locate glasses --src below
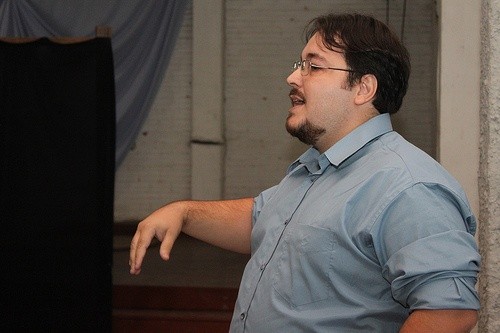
[293,60,365,76]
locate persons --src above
[128,13,482,333]
